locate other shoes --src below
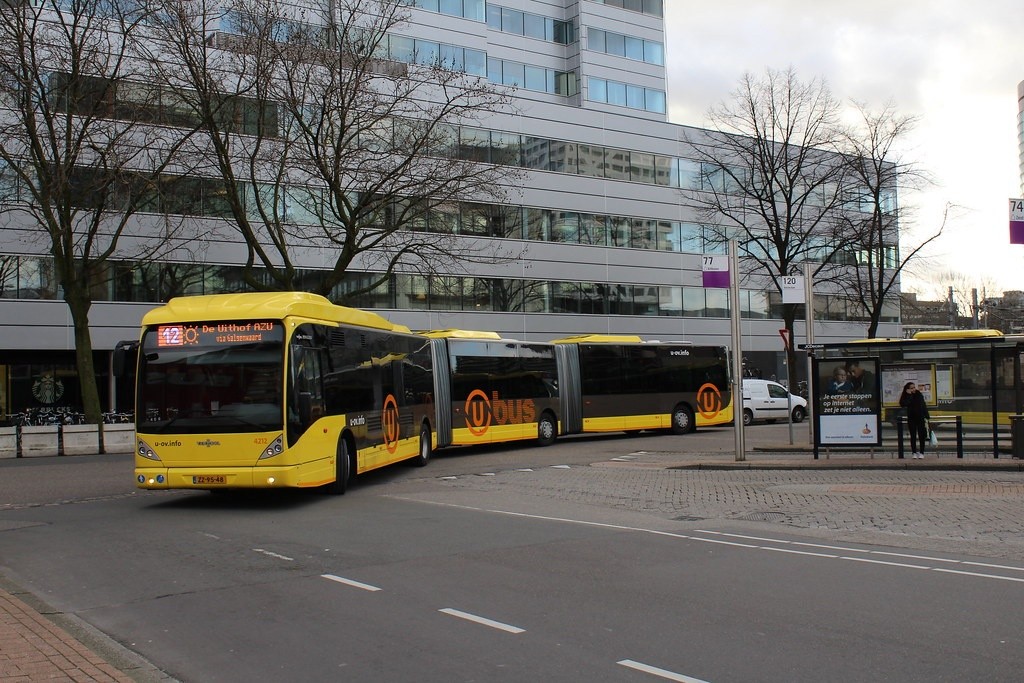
[918,453,924,458]
[912,453,917,458]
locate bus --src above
[848,329,1024,428]
[113,292,736,495]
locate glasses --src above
[838,374,846,376]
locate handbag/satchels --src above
[928,421,938,448]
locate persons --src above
[845,361,876,414]
[899,382,930,459]
[828,365,852,395]
[771,374,776,382]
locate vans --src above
[742,379,808,425]
